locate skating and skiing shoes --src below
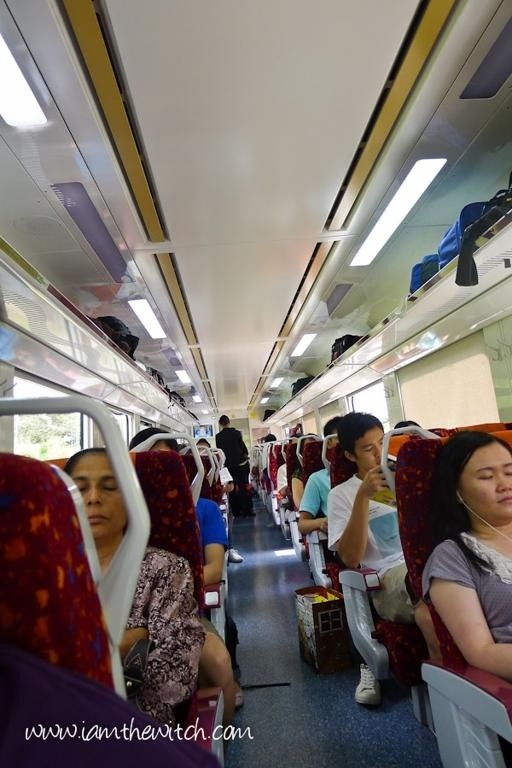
[228,548,244,563]
[353,660,386,707]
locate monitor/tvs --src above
[193,424,214,439]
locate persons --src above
[51,408,512,744]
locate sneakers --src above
[233,511,257,517]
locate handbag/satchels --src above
[293,584,347,675]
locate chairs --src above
[0,395,512,768]
